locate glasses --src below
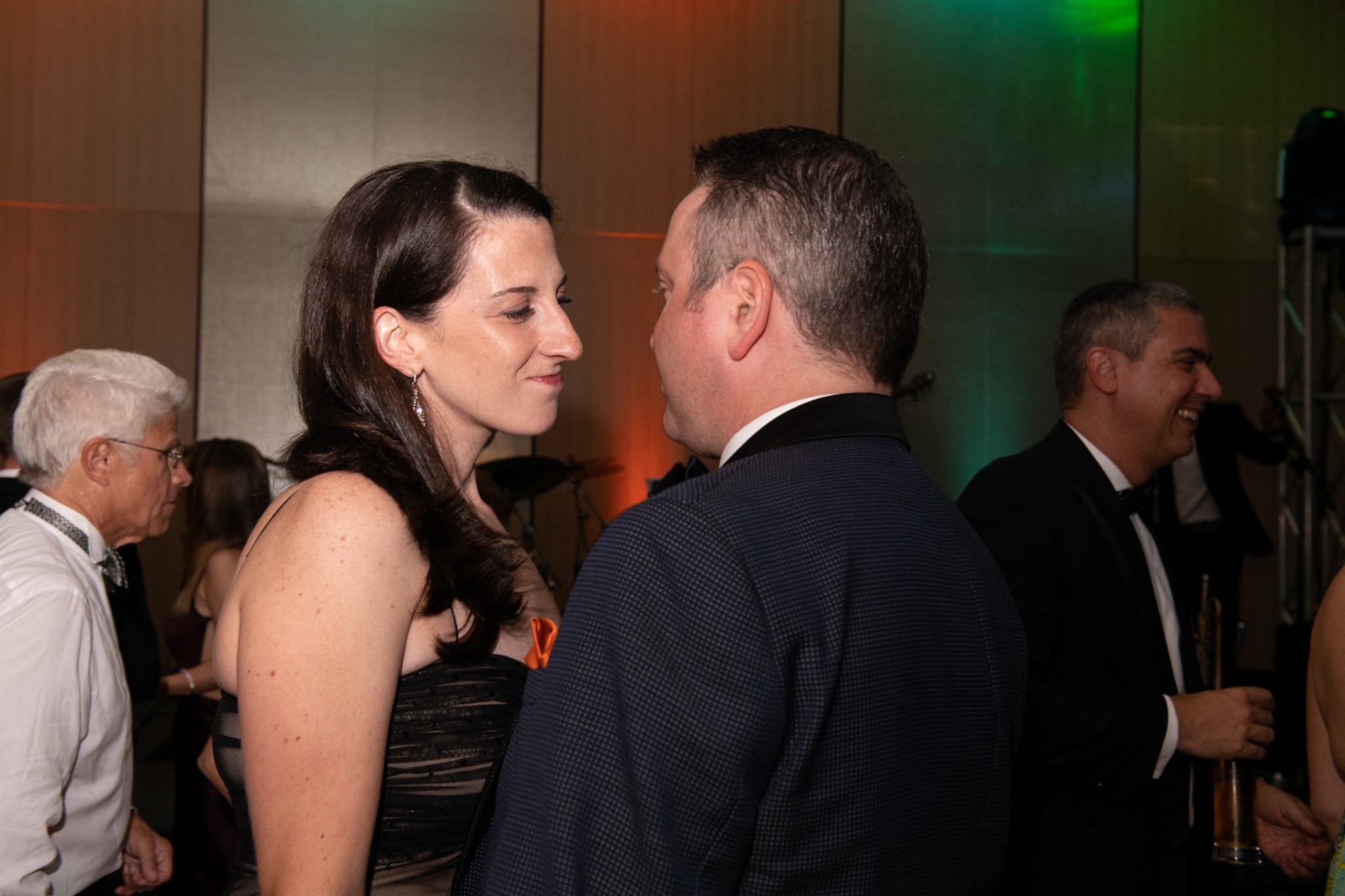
[107,436,184,469]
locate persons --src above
[466,122,1002,896]
[177,158,588,896]
[958,278,1345,896]
[0,350,190,895]
[157,437,274,895]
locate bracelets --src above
[182,666,196,692]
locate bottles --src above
[1211,756,1264,866]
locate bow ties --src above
[647,456,710,499]
[16,497,128,588]
[1116,479,1156,515]
